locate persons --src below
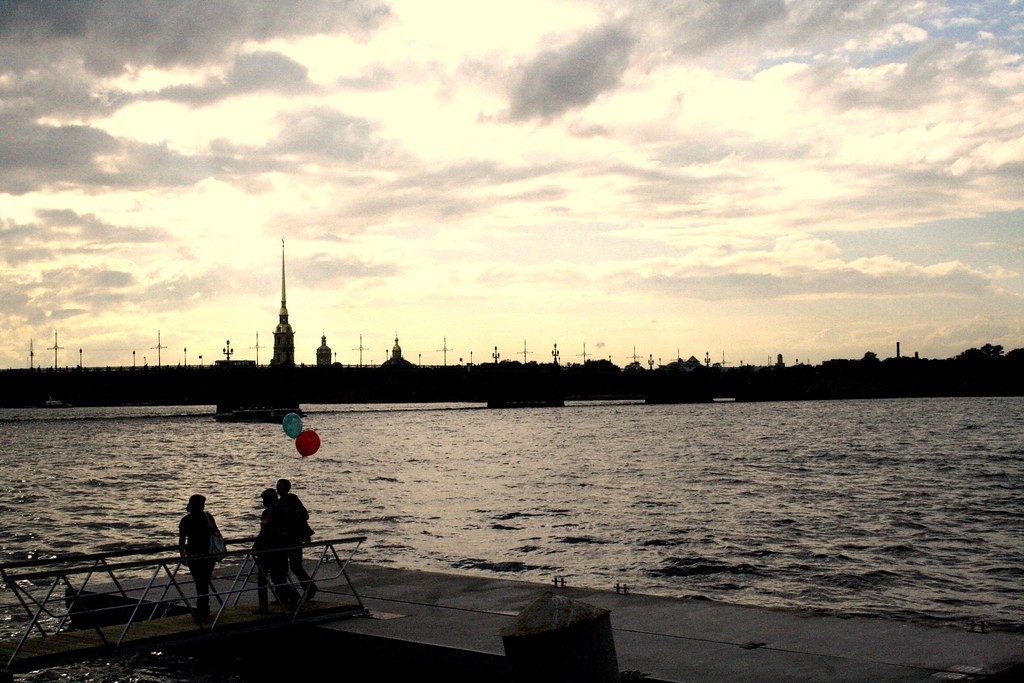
[179,494,220,616]
[251,479,318,615]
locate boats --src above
[213,338,306,422]
[45,397,72,408]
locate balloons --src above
[283,413,303,439]
[295,430,321,457]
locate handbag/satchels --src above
[209,531,226,561]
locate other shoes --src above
[302,589,319,600]
[271,599,289,605]
[254,609,269,615]
